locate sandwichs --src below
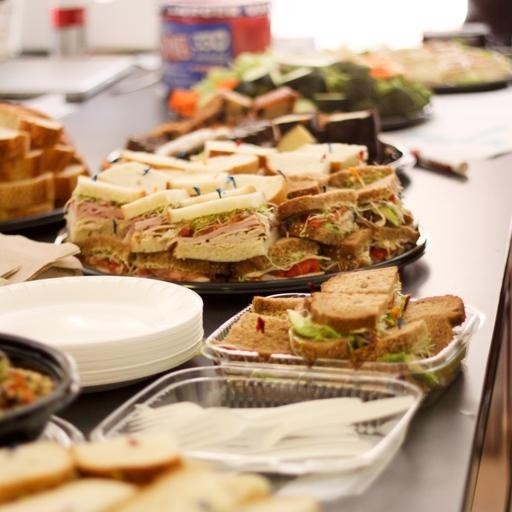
[0,98,465,377]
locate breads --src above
[0,430,323,511]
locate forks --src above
[125,394,415,460]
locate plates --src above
[171,104,428,130]
[121,137,415,177]
[0,275,204,386]
[353,44,511,94]
[51,229,428,290]
[0,200,66,236]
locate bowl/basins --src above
[0,333,81,449]
